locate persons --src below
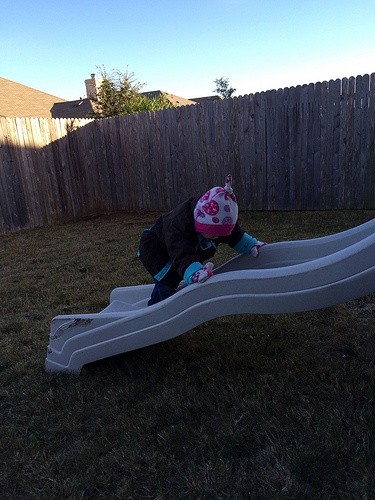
[138,173,268,307]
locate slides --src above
[44,215,375,378]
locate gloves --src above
[191,261,215,283]
[250,240,268,257]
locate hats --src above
[193,174,239,236]
